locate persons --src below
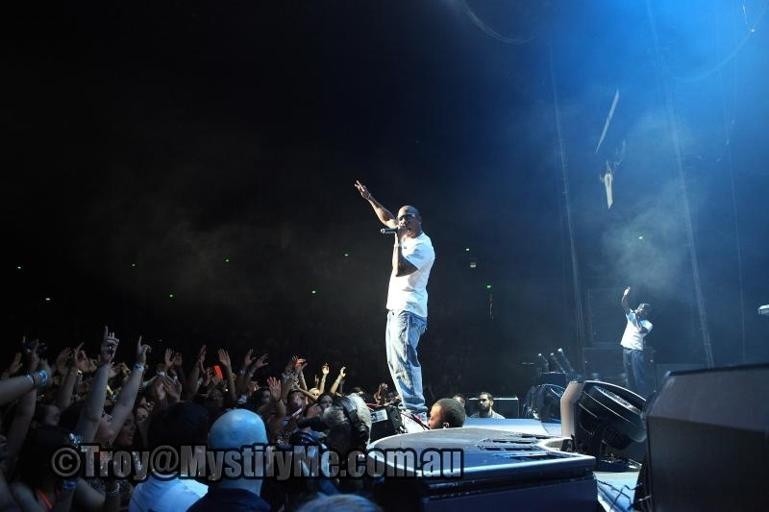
[354,179,436,423]
[1,320,507,510]
[620,286,654,394]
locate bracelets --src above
[396,243,404,248]
[365,192,372,200]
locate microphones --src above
[380,227,398,234]
[631,311,635,314]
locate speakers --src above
[645,361,769,512]
